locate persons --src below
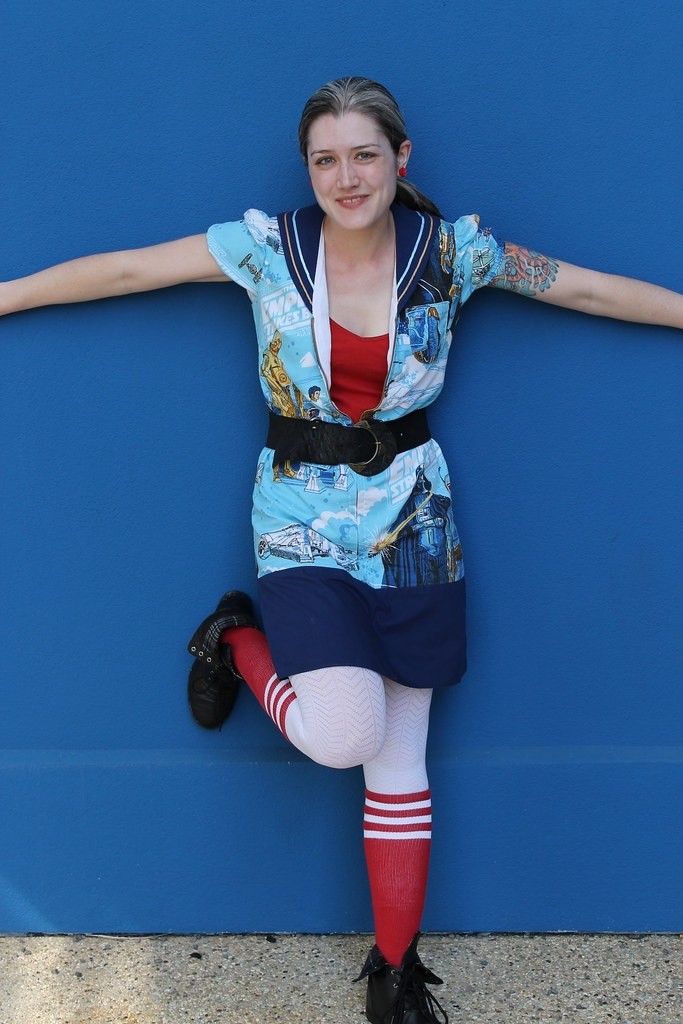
[0,76,682,1024]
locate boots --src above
[350,930,450,1024]
[188,589,264,732]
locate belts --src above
[264,408,431,476]
